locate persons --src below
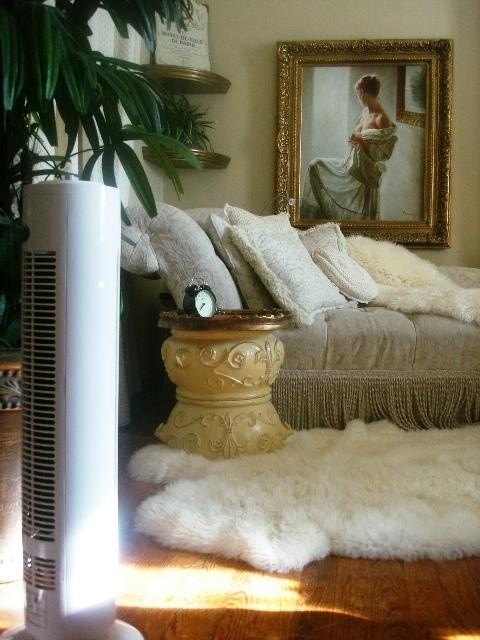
[300,75,398,221]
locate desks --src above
[149,305,300,458]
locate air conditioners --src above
[1,180,154,640]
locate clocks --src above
[181,279,219,318]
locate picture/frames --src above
[271,34,455,254]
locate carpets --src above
[123,419,478,574]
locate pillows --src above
[206,204,376,325]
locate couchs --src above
[118,205,478,431]
